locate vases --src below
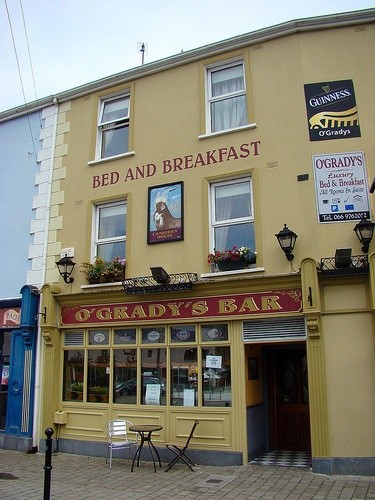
[88,272,124,285]
[217,261,247,270]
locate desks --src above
[129,425,163,473]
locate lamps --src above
[353,214,375,253]
[34,306,47,323]
[335,249,352,268]
[55,253,76,283]
[275,224,298,261]
[150,267,170,284]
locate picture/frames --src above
[147,181,184,245]
[248,357,259,380]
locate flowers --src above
[79,256,126,282]
[208,245,257,264]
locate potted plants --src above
[68,382,109,404]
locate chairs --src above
[105,419,140,470]
[165,420,199,473]
